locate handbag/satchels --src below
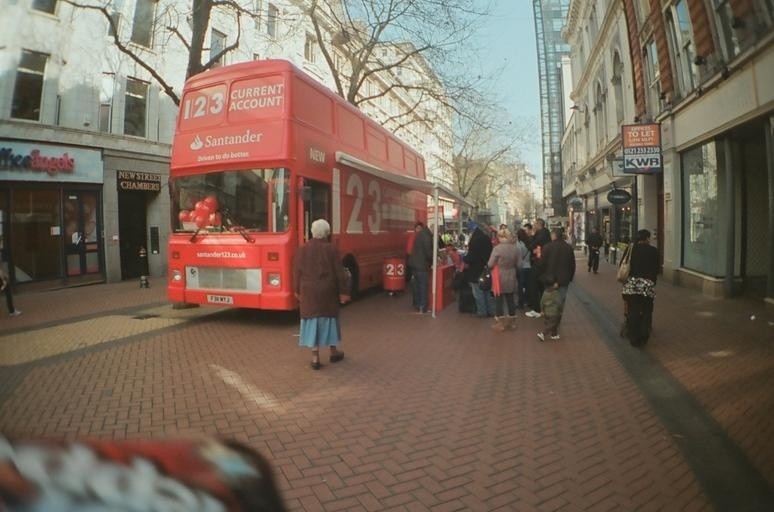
[616,263,631,282]
[478,267,491,290]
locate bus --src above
[165,58,427,315]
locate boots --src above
[504,314,517,330]
[490,315,505,331]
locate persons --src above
[408,217,576,341]
[619,230,659,345]
[585,226,602,272]
[294,218,349,368]
[1,238,21,318]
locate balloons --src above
[176,196,224,229]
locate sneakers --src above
[330,352,343,362]
[524,310,542,319]
[8,309,22,318]
[311,360,320,370]
